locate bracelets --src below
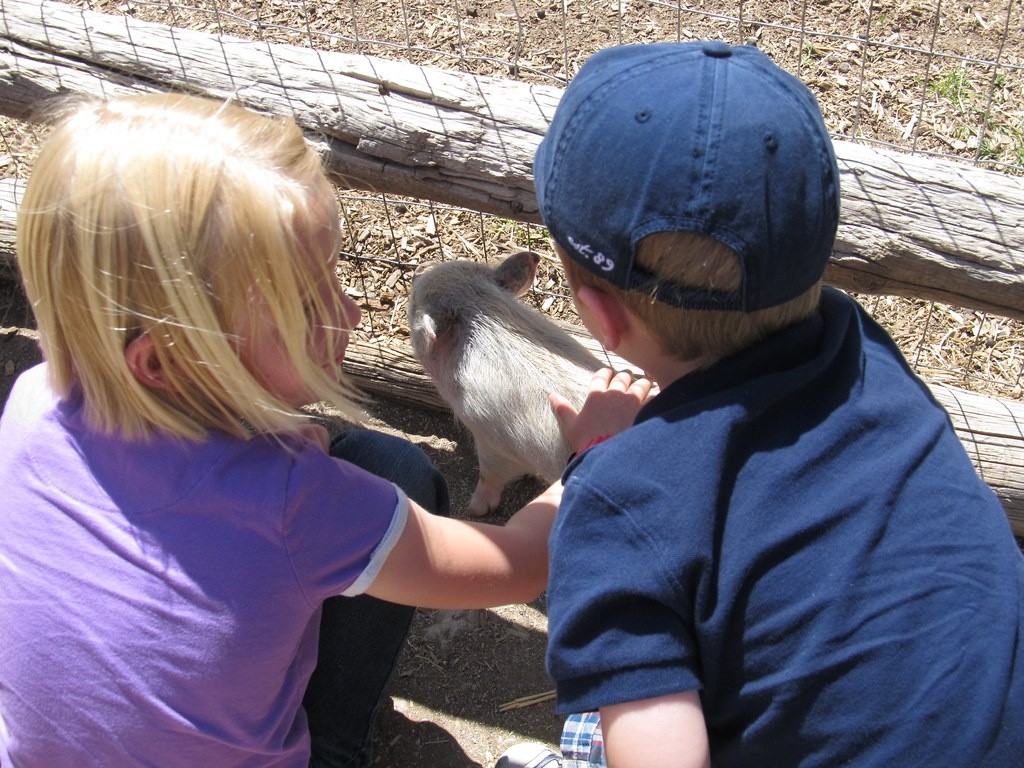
[567,433,614,459]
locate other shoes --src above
[495,742,562,768]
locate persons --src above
[496,40,1024,768]
[0,90,659,768]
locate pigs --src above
[406,252,664,517]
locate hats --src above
[532,39,839,311]
